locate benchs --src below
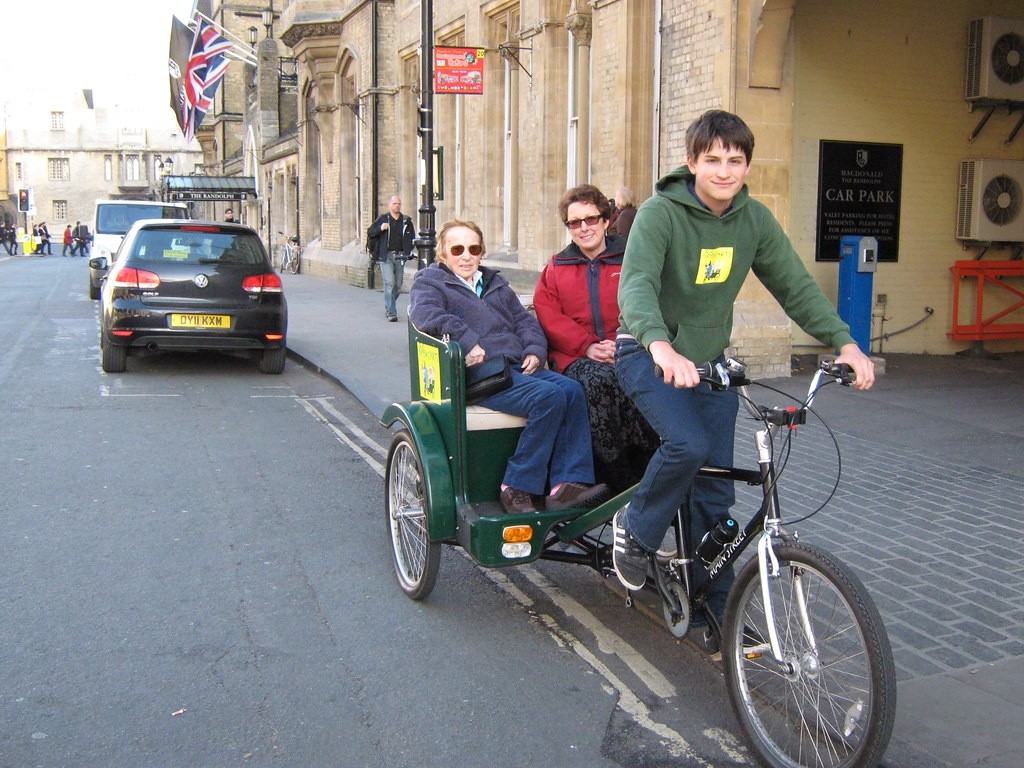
[464,404,531,432]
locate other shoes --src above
[71,254,77,257]
[62,255,67,257]
[34,252,46,254]
[81,255,86,257]
[48,253,53,255]
[10,253,18,256]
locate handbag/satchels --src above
[34,233,43,244]
[465,353,513,399]
[366,213,390,259]
[38,227,47,240]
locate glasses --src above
[451,245,483,256]
[566,214,603,229]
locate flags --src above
[169,13,233,144]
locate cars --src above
[88,218,288,374]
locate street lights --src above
[164,157,173,203]
[158,162,165,203]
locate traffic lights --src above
[20,188,29,212]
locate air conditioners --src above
[966,13,1024,103]
[957,158,1024,241]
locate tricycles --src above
[379,308,899,768]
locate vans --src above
[88,198,191,301]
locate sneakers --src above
[703,624,772,662]
[389,316,397,322]
[500,486,536,514]
[613,502,648,591]
[545,482,608,510]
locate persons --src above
[224,208,240,223]
[0,221,89,257]
[368,196,416,323]
[611,110,875,663]
[407,216,597,514]
[531,185,662,498]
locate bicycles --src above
[278,231,301,275]
